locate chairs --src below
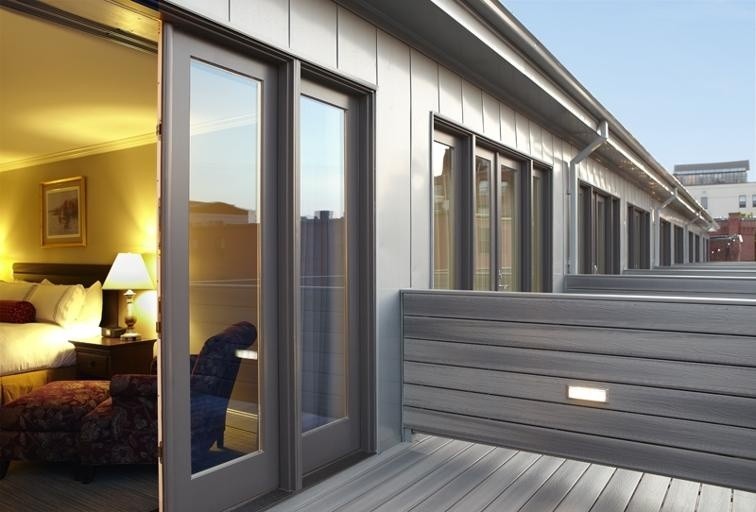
[78,322,254,485]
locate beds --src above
[0,263,121,413]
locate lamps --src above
[102,252,154,341]
[566,385,609,404]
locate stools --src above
[0,380,112,480]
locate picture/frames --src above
[38,175,87,249]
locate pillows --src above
[0,279,105,327]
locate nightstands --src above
[68,337,156,381]
